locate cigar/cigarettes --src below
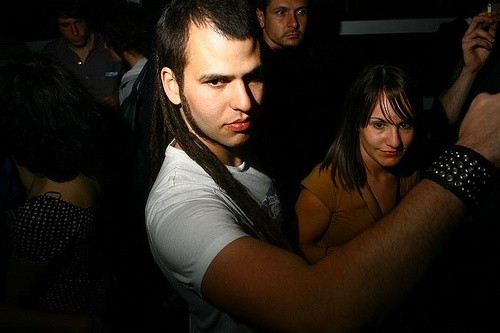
[487,3,492,18]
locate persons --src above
[0,0,500,333]
